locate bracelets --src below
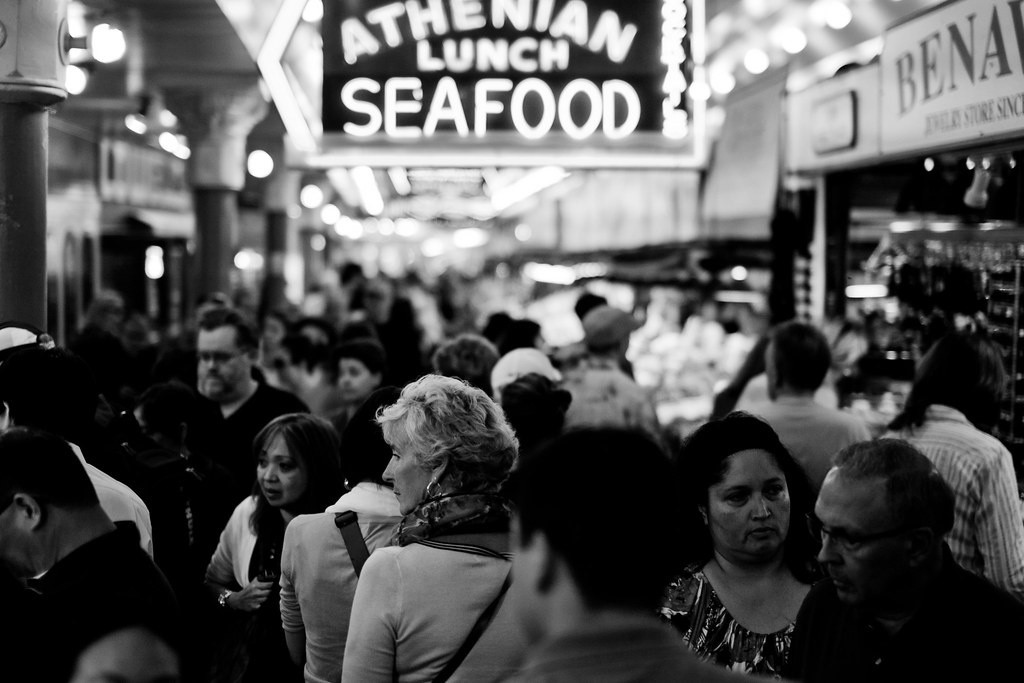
[218,590,233,607]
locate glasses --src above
[804,510,902,552]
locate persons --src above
[0,263,1024,683]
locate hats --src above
[583,304,642,348]
[489,345,565,402]
